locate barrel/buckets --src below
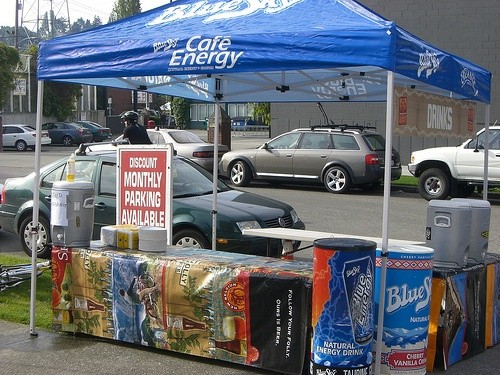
[50,180,95,247]
[426,199,472,269]
[451,196,492,265]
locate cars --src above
[2,124,52,151]
[75,121,112,142]
[0,151,305,259]
[113,127,228,173]
[41,122,93,146]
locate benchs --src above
[337,142,356,148]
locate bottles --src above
[209,339,247,357]
[75,296,104,311]
[166,315,206,332]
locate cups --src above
[223,316,246,340]
[66,159,75,182]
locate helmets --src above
[120,111,139,119]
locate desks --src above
[426,253,500,372]
[242,226,427,258]
[50,238,314,375]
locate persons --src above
[120,110,154,145]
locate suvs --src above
[217,124,402,193]
[407,124,500,201]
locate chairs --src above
[319,141,328,148]
[303,138,312,148]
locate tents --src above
[29,0,492,375]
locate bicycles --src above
[133,274,159,320]
